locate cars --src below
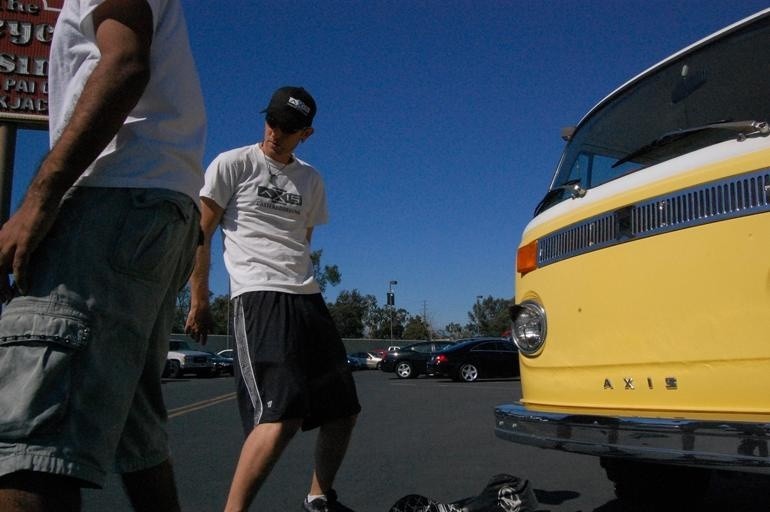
[346,337,520,383]
[164,337,234,378]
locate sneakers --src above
[302,490,357,512]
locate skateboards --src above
[389,472,539,512]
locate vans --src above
[493,6,770,502]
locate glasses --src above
[264,114,306,134]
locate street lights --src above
[386,278,398,346]
[476,293,483,337]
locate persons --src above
[0,0,209,512]
[186,87,363,511]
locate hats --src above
[260,86,317,125]
